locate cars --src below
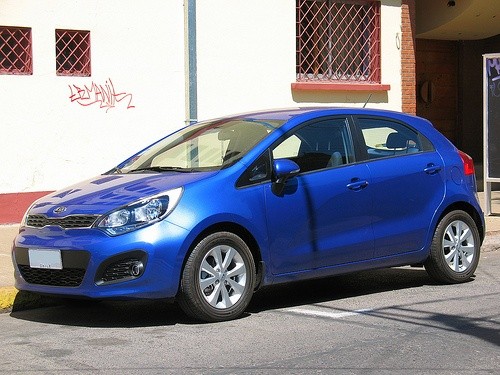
[12,107,487,323]
